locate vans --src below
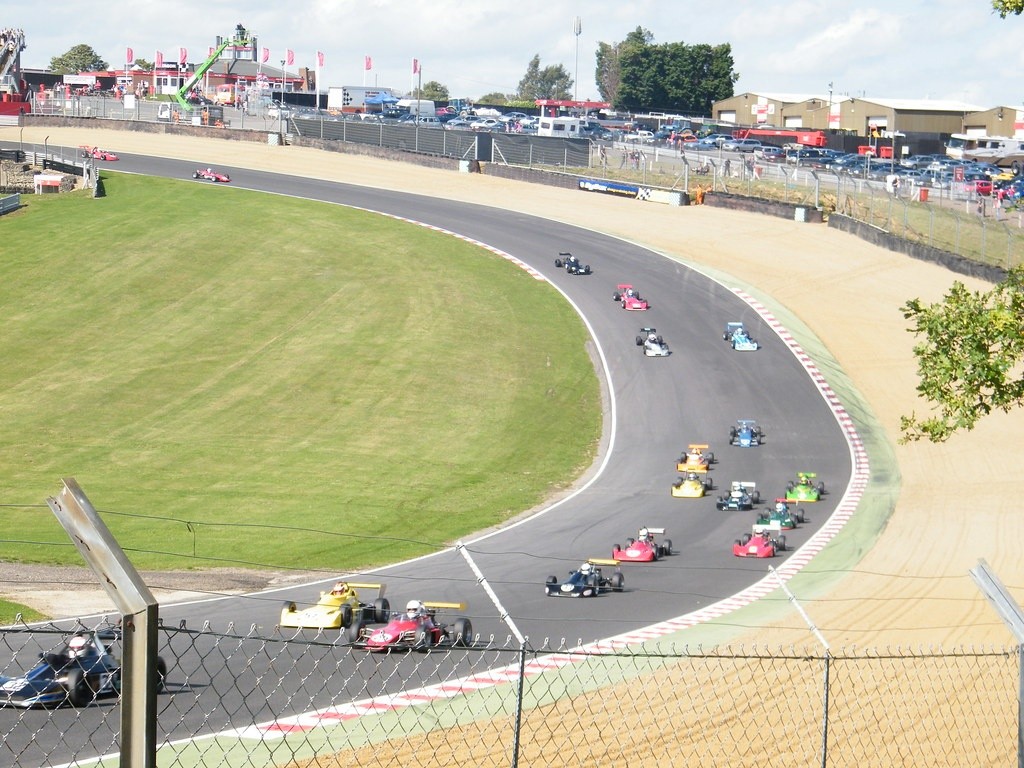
[395,99,435,118]
[723,139,761,152]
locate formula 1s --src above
[281,583,392,631]
[636,327,671,356]
[670,466,713,498]
[192,169,231,182]
[545,557,626,597]
[756,497,807,529]
[716,481,760,510]
[79,145,119,161]
[1,626,166,707]
[611,526,673,562]
[677,443,714,472]
[556,251,591,276]
[612,283,647,310]
[346,600,474,653]
[727,419,762,447]
[785,472,826,501]
[731,523,787,559]
[722,322,758,351]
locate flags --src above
[318,51,324,68]
[157,52,163,67]
[413,58,418,73]
[365,56,371,69]
[262,48,269,62]
[287,50,294,65]
[210,48,214,57]
[180,48,187,64]
[127,48,133,63]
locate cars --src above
[436,108,540,134]
[268,99,291,111]
[185,95,214,106]
[378,108,404,120]
[394,114,422,124]
[212,96,234,107]
[579,120,1024,201]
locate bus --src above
[218,84,242,108]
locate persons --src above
[332,582,352,597]
[580,563,594,576]
[628,288,634,295]
[670,418,812,537]
[638,528,649,543]
[570,256,575,262]
[207,168,212,175]
[203,107,208,125]
[648,332,657,341]
[723,158,731,176]
[736,328,745,334]
[67,637,90,658]
[893,177,899,198]
[172,109,179,125]
[405,600,423,621]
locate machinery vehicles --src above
[154,28,254,127]
[731,129,828,148]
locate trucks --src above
[942,134,1024,166]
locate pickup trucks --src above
[786,149,832,168]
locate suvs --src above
[753,146,783,158]
[403,115,441,129]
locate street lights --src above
[280,60,287,105]
[891,130,906,175]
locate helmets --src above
[692,449,697,454]
[742,425,748,432]
[800,476,808,484]
[68,636,89,657]
[755,528,763,537]
[207,168,212,173]
[776,503,783,512]
[649,334,656,341]
[639,528,648,541]
[732,481,741,491]
[333,581,349,596]
[737,328,743,334]
[406,600,421,618]
[688,472,697,480]
[570,256,576,262]
[581,563,591,575]
[628,290,634,296]
[97,148,102,153]
